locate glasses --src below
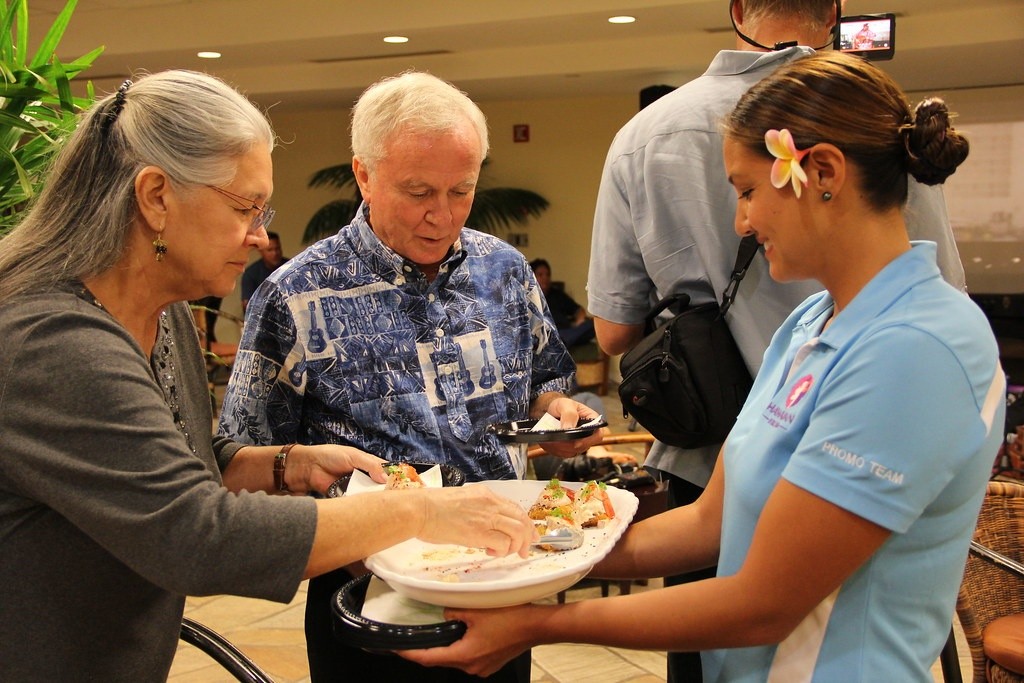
[205,183,275,233]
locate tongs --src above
[526,528,582,550]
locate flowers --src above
[764,128,810,199]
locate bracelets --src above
[273,444,295,493]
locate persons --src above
[241,232,290,313]
[0,70,543,683]
[531,258,594,350]
[586,0,967,683]
[393,50,1007,683]
[855,23,876,48]
[217,67,611,683]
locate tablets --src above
[833,13,896,61]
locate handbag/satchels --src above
[618,295,755,448]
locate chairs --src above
[189,305,244,419]
[551,281,609,397]
[953,481,1024,683]
[527,433,658,604]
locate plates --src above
[330,573,468,656]
[487,417,608,443]
[327,462,465,508]
[363,479,639,607]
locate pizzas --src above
[528,479,576,520]
[385,462,422,491]
[572,479,615,528]
